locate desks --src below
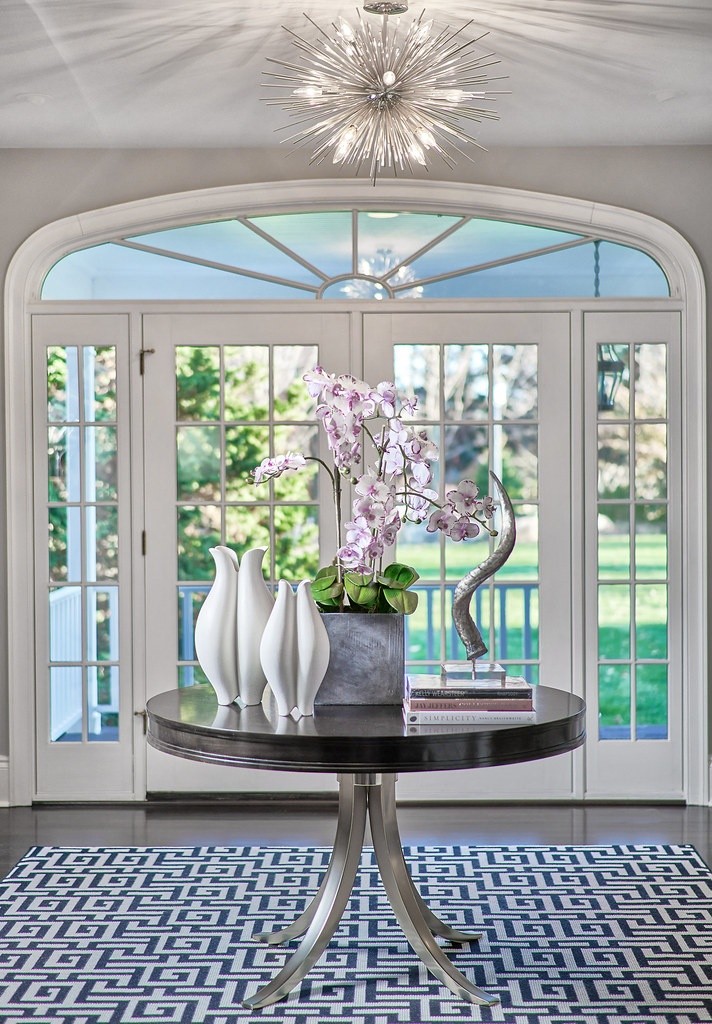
[145,683,586,1011]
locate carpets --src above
[0,843,712,1024]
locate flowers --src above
[250,364,499,615]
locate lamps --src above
[259,0,512,187]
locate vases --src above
[259,578,330,717]
[314,613,407,705]
[194,546,275,705]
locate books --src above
[401,674,536,726]
